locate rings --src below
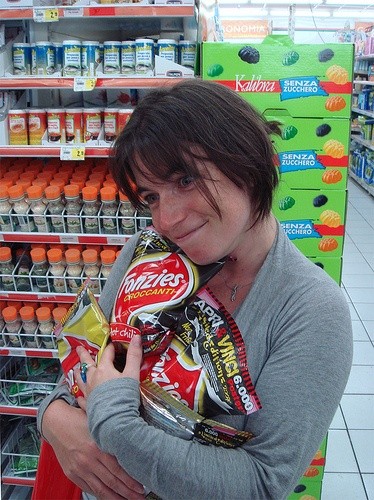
[78,362,94,384]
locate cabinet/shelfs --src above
[0,0,374,500]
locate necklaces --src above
[219,273,253,301]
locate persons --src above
[37,79,354,500]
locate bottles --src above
[0,156,152,235]
[0,240,124,295]
[0,300,74,349]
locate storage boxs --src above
[199,40,355,119]
[306,257,343,289]
[285,481,323,500]
[301,431,328,481]
[263,116,351,190]
[270,188,349,257]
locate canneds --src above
[12,38,198,76]
[7,107,135,144]
